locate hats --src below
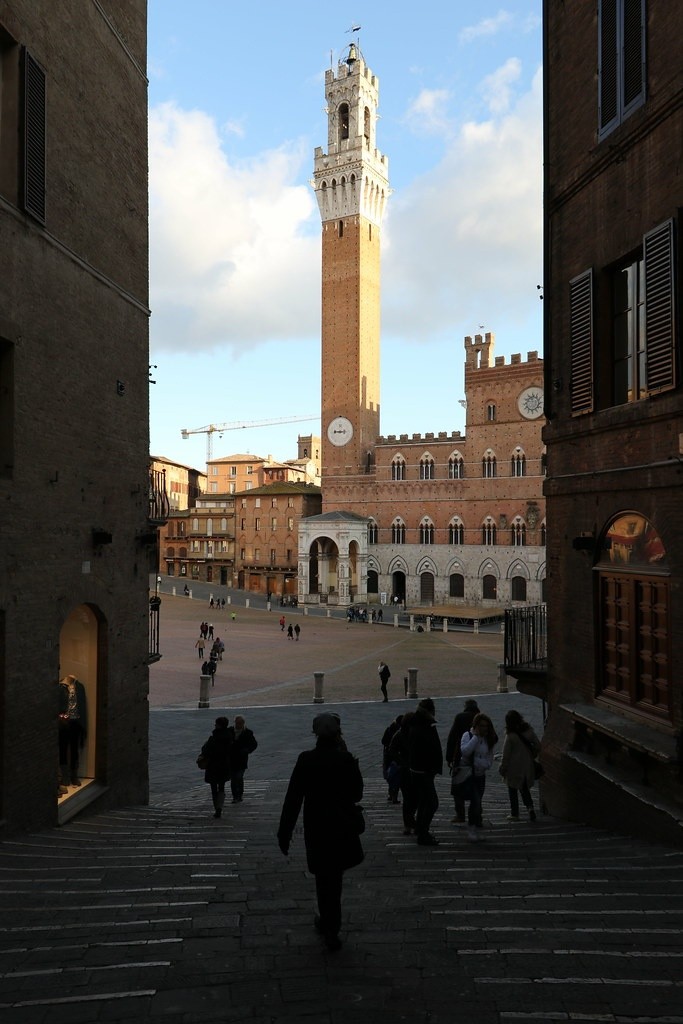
[313,710,341,736]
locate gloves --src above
[278,837,290,855]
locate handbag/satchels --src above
[355,803,366,834]
[534,758,546,780]
[456,773,480,801]
[196,754,205,768]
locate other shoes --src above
[313,917,342,954]
[417,836,439,845]
[451,816,466,822]
[71,778,81,786]
[61,777,70,786]
[387,797,401,804]
[231,797,243,803]
[507,814,518,821]
[213,807,223,818]
[528,809,536,823]
[58,789,68,798]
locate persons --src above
[276,711,364,939]
[266,591,300,642]
[430,612,436,627]
[377,662,389,702]
[184,584,237,687]
[380,698,540,845]
[345,593,404,622]
[203,716,232,819]
[59,675,88,796]
[226,715,258,804]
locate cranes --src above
[181,414,322,493]
